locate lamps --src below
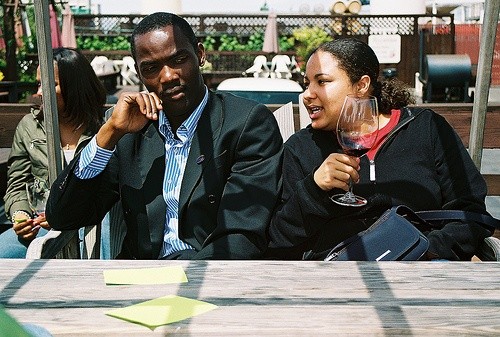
[418,28,474,103]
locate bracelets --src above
[12,211,31,225]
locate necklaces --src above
[67,122,84,150]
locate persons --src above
[264,36,495,261]
[0,47,107,259]
[44,12,285,260]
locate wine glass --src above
[331,94,379,206]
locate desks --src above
[0,257,500,337]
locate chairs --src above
[90,55,139,85]
[245,54,294,80]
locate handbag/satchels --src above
[324,210,430,261]
